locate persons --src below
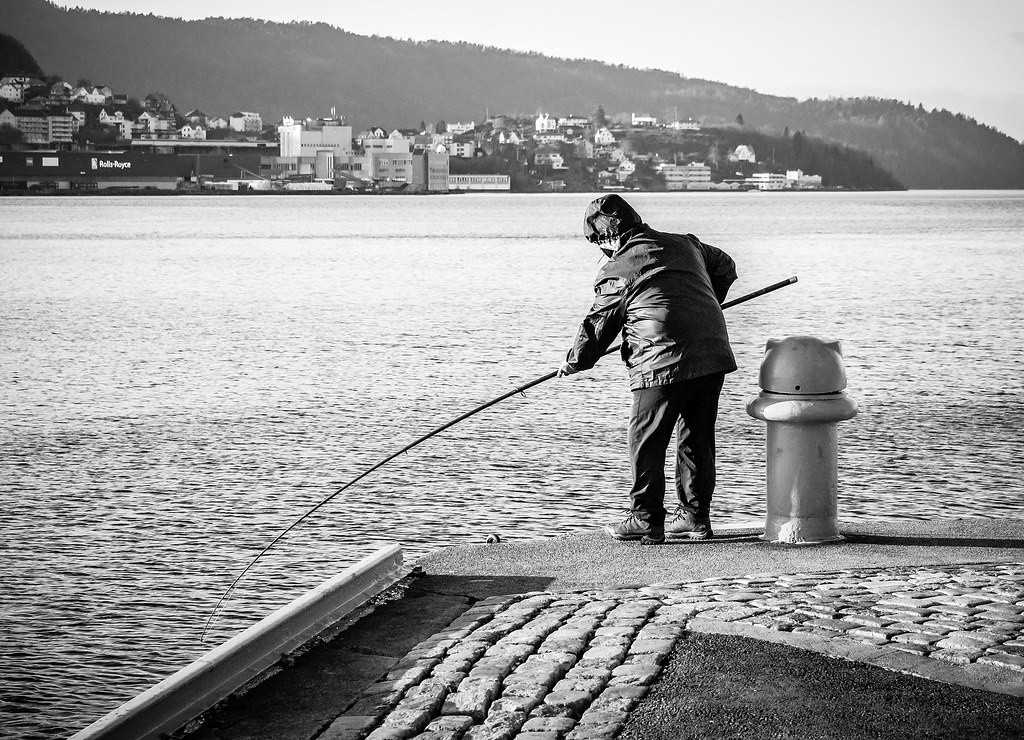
[557,193,737,545]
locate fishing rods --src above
[200,275,799,649]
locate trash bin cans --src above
[745,335,858,544]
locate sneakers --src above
[606,509,665,545]
[663,507,713,540]
